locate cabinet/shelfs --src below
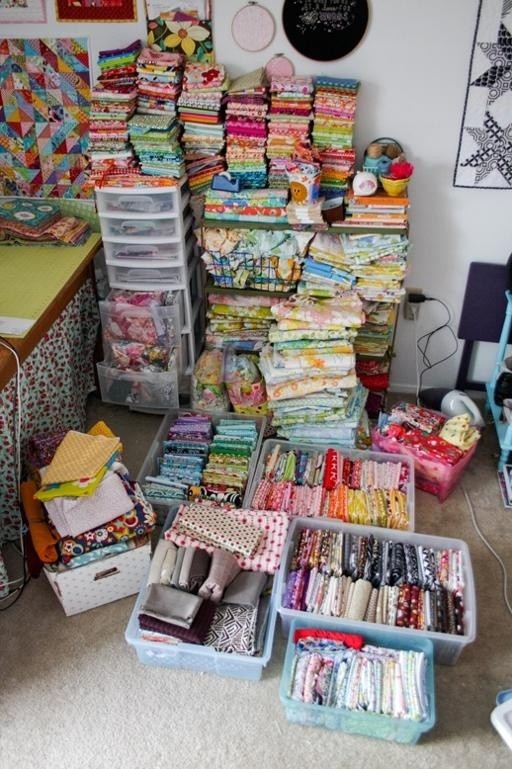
[481,288,512,474]
[96,173,412,419]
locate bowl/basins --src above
[378,174,410,197]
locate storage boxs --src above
[137,408,268,510]
[275,518,482,665]
[247,437,419,532]
[122,504,293,681]
[371,404,481,503]
[279,616,439,745]
[22,470,151,617]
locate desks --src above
[0,232,101,396]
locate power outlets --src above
[403,287,422,319]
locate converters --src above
[407,292,425,303]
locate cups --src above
[285,159,323,206]
[320,195,346,224]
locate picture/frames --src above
[1,0,212,24]
